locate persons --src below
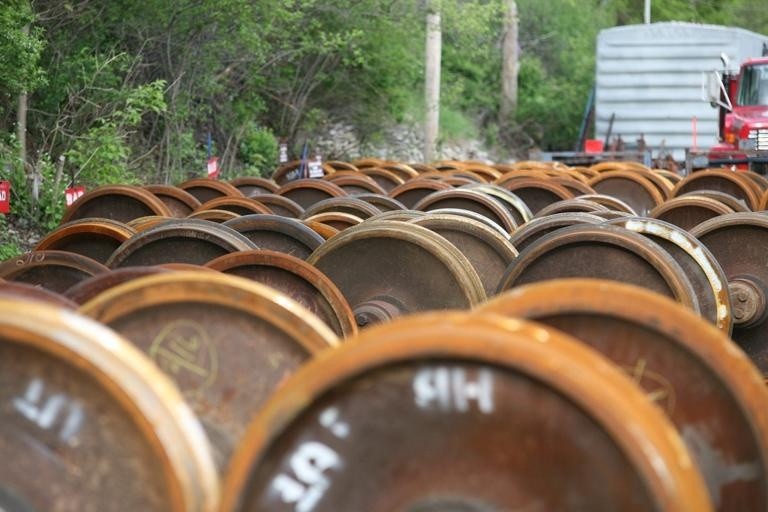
[651,155,686,179]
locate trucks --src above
[702,51,768,173]
